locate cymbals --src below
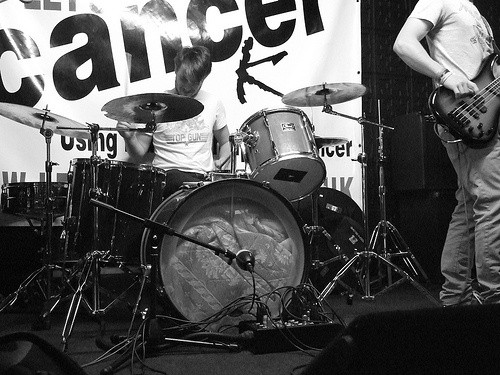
[314,137,348,147]
[282,83,366,107]
[101,92,204,123]
[0,102,92,139]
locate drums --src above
[62,158,166,268]
[141,177,310,333]
[238,106,326,201]
[1,182,71,215]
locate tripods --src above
[0,117,241,375]
[302,92,445,318]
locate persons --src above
[392,0,500,305]
[115,46,233,203]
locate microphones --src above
[236,249,255,272]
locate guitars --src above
[429,53,500,149]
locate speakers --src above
[301,302,500,375]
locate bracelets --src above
[435,68,452,85]
